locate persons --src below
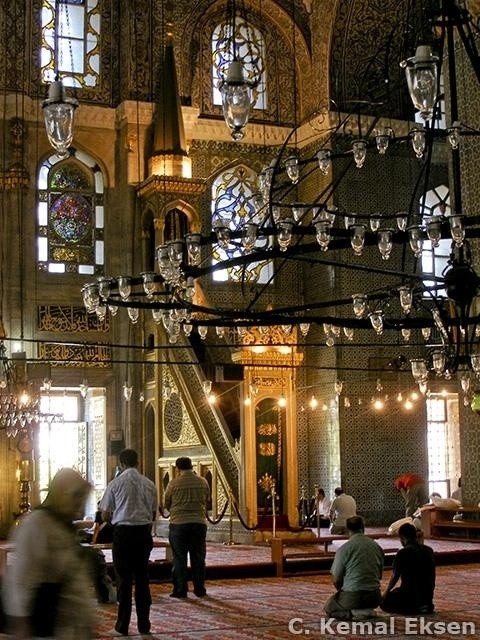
[80,498,116,544]
[452,478,462,501]
[328,486,357,537]
[71,516,94,543]
[164,456,210,599]
[309,488,332,528]
[378,523,437,614]
[1,467,98,640]
[99,449,157,636]
[323,516,385,623]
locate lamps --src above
[39,0,444,155]
[0,337,51,439]
[78,0,478,395]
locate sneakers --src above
[422,603,434,614]
[169,591,188,599]
[108,628,123,636]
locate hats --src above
[347,517,365,530]
